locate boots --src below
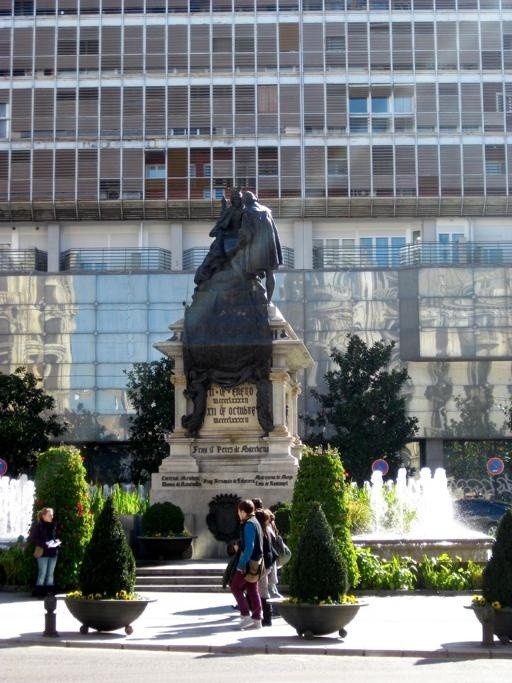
[262,598,272,626]
[36,585,56,600]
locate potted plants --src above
[463,510,512,645]
[267,503,368,641]
[135,501,198,560]
[55,495,158,635]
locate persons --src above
[193,190,247,286]
[236,192,284,305]
[222,498,283,630]
[30,508,60,600]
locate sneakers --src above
[240,617,262,631]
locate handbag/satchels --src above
[274,534,291,567]
[33,546,44,559]
[242,555,265,583]
